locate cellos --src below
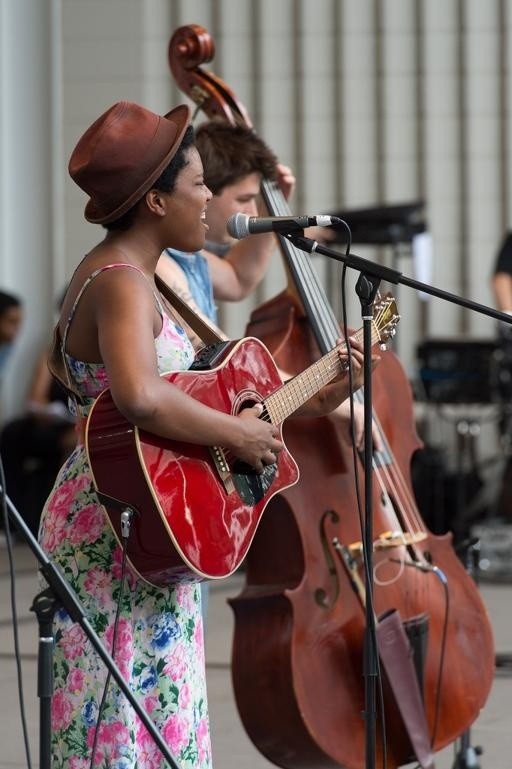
[165,22,497,768]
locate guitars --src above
[84,288,402,590]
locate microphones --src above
[226,211,335,238]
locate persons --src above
[158,123,385,456]
[491,230,512,523]
[0,291,68,542]
[39,103,381,769]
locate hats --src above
[64,98,193,226]
